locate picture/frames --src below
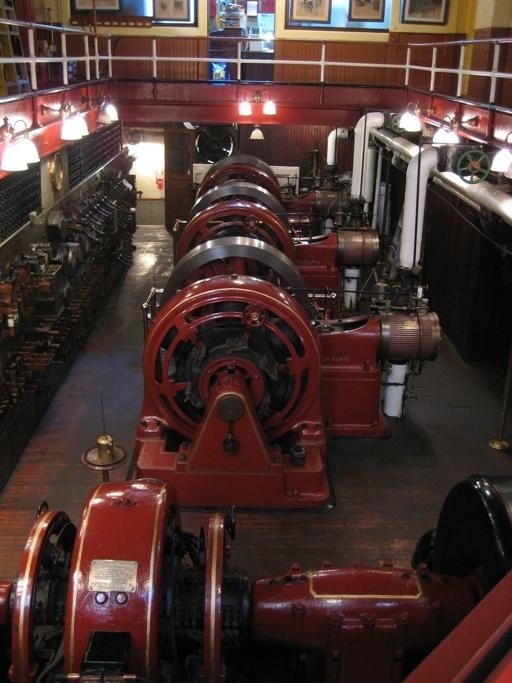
[347,0,385,22]
[70,0,123,14]
[152,0,192,22]
[289,0,332,23]
[397,0,450,26]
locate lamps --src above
[236,86,277,117]
[395,95,511,179]
[1,86,121,173]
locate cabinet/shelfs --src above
[209,26,249,80]
